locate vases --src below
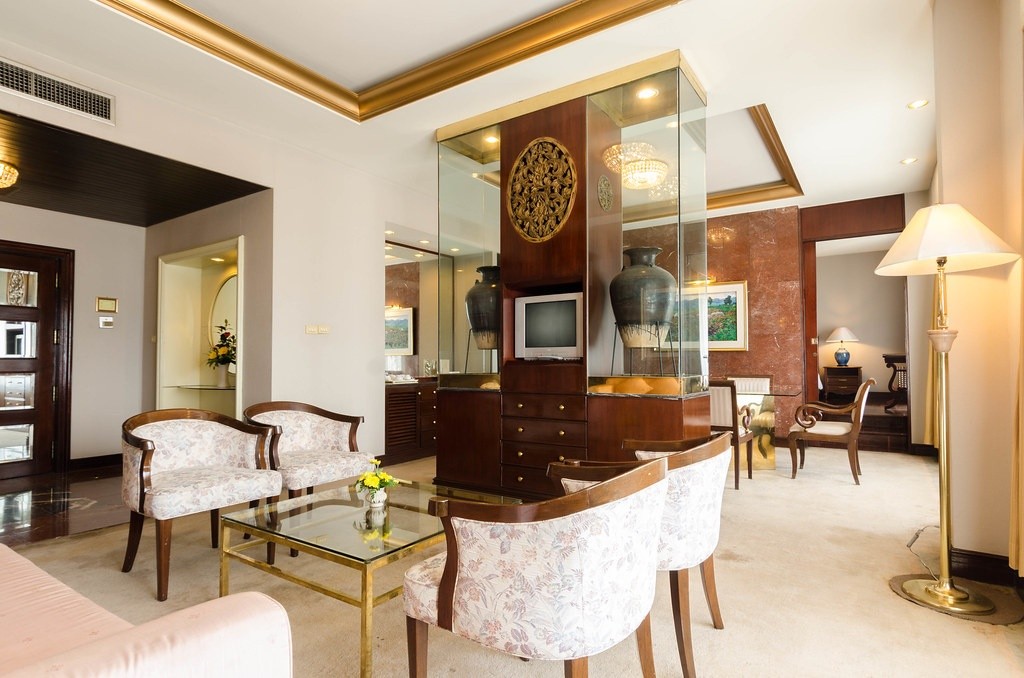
[366,487,388,506]
[215,362,231,388]
[365,507,385,528]
[609,246,677,348]
[466,265,500,350]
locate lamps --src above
[602,142,657,173]
[825,327,860,367]
[0,160,20,189]
[873,203,1023,616]
[620,160,668,190]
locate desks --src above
[725,389,802,472]
[882,354,908,413]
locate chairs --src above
[787,377,877,485]
[748,395,775,458]
[706,374,774,490]
[122,400,732,678]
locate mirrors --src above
[384,240,454,378]
[207,275,237,376]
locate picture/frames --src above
[653,280,749,352]
[384,307,414,355]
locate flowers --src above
[203,320,236,370]
[352,521,392,553]
[349,457,397,499]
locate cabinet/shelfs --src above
[382,377,437,466]
[430,388,710,503]
[823,366,862,403]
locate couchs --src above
[0,541,294,678]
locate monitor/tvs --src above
[515,292,584,361]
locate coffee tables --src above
[219,478,522,678]
